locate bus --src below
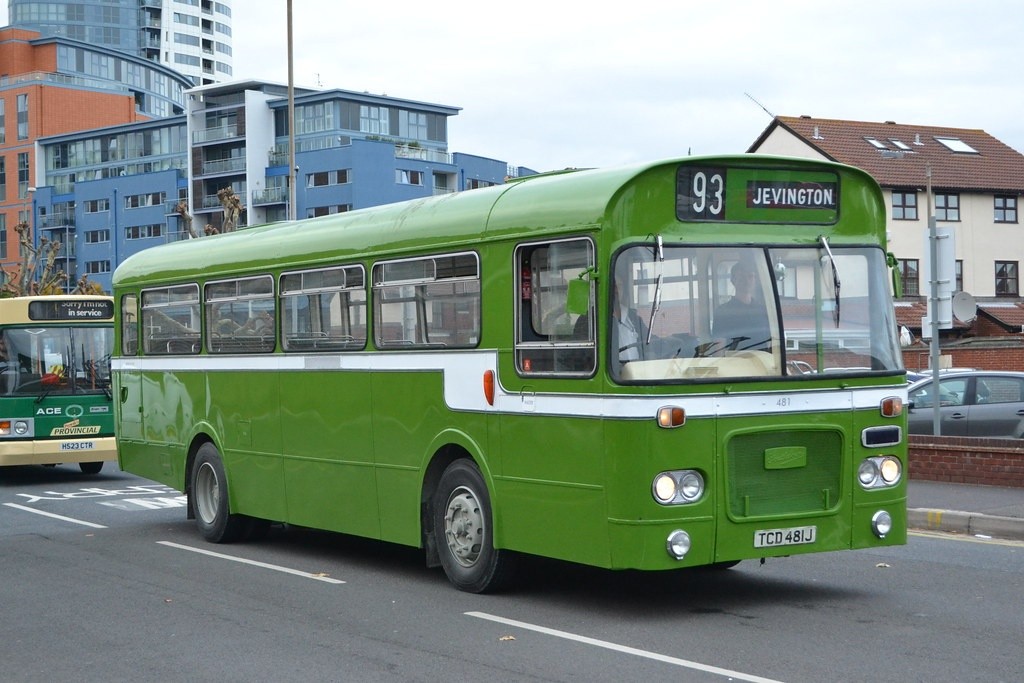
[111,153,909,596]
[0,295,138,474]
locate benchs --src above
[126,330,448,352]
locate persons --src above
[0,335,28,374]
[712,261,771,352]
[571,271,670,372]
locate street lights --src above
[24,187,36,222]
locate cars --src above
[804,365,1024,440]
